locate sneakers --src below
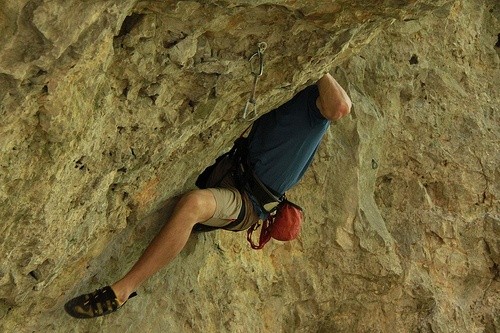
[63,287,137,318]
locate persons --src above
[64,73,351,319]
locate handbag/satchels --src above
[194,154,233,190]
[269,200,302,241]
